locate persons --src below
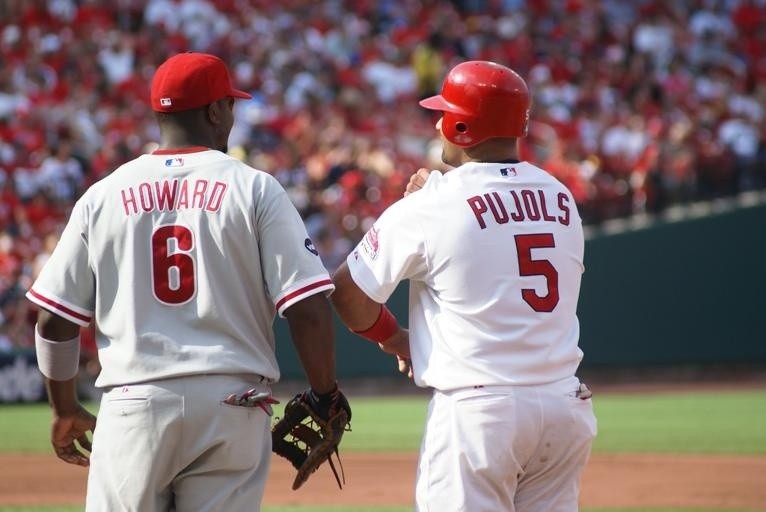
[23,51,352,512]
[0,0,766,350]
[327,60,599,512]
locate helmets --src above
[419,61,530,148]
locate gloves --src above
[224,388,280,416]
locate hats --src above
[151,52,252,113]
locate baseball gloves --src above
[271,379,351,490]
